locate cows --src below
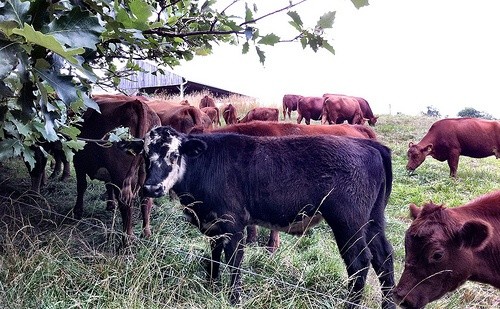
[394,190,500,309]
[405,118,500,179]
[17,94,398,309]
